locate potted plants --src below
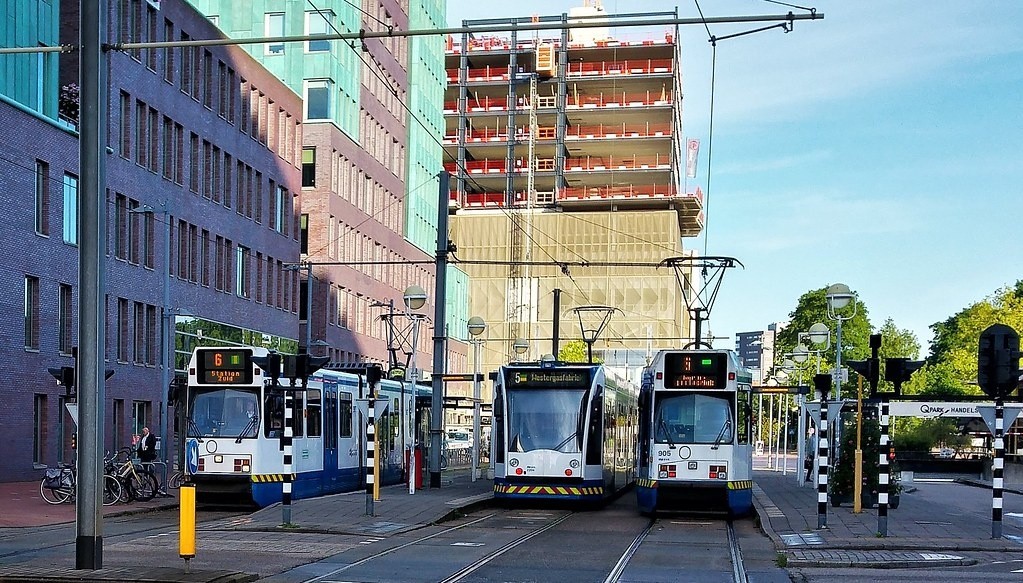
[888,482,904,509]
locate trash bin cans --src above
[406,443,424,490]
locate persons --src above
[243,399,256,420]
[804,429,822,482]
[132,433,141,446]
[138,427,157,470]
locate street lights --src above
[822,282,857,476]
[401,284,430,495]
[465,316,490,484]
[127,200,172,495]
[798,323,831,494]
[761,345,810,477]
[368,299,394,368]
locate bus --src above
[632,255,755,521]
[492,305,638,512]
[166,313,435,513]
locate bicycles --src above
[39,445,188,506]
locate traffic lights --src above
[845,358,879,384]
[250,352,281,375]
[996,349,1023,388]
[884,358,926,385]
[295,351,330,381]
[47,366,75,396]
